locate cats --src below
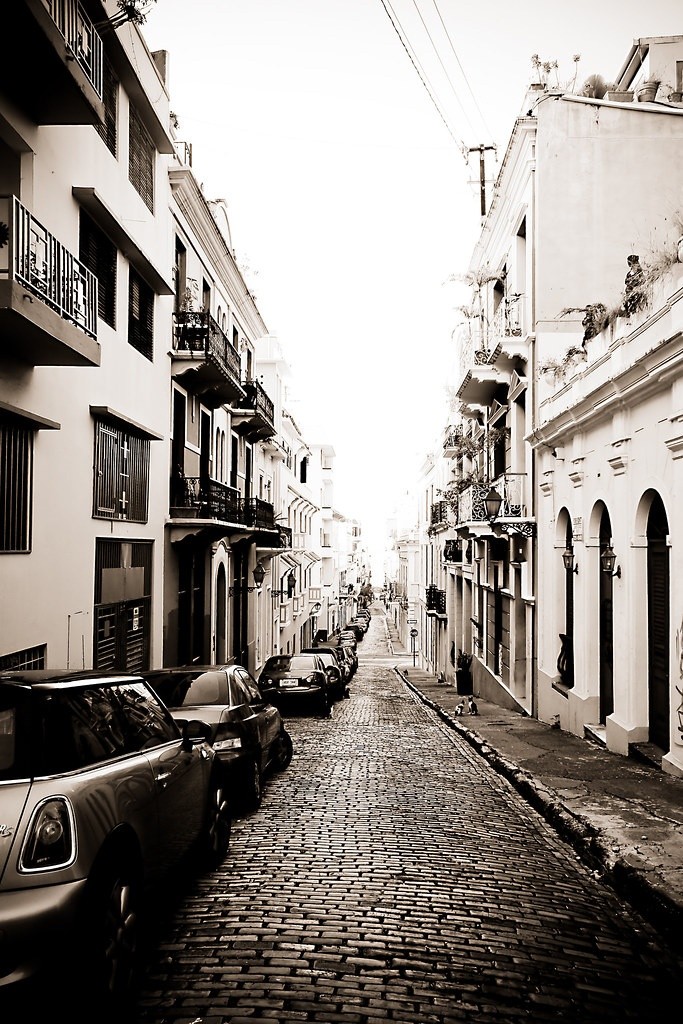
[454,696,478,716]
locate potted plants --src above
[453,649,474,697]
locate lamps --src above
[271,572,296,598]
[600,545,622,579]
[309,601,321,615]
[229,561,266,597]
[563,546,578,573]
[485,488,537,541]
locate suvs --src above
[0,669,230,1015]
[301,647,346,701]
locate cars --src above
[315,654,340,703]
[258,654,334,715]
[329,608,371,682]
[140,665,293,811]
